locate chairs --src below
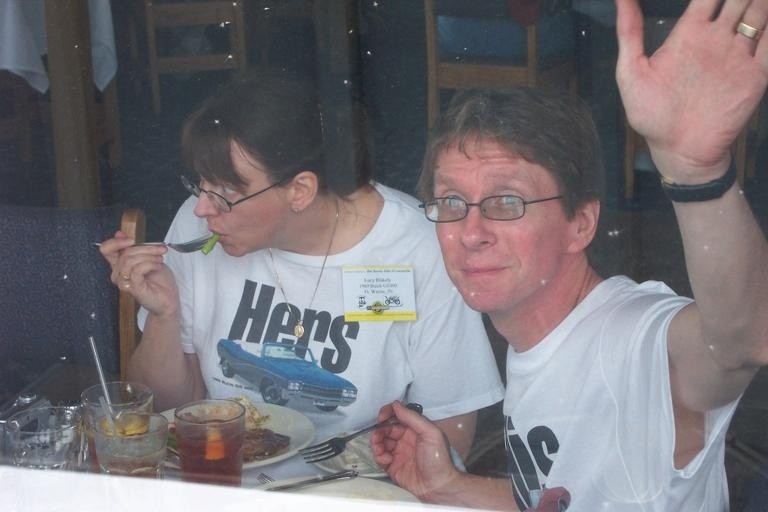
[425,0,580,139]
[621,18,764,202]
[128,0,247,119]
[0,201,148,407]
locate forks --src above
[298,402,423,464]
[91,234,215,253]
[256,474,277,484]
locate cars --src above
[217,337,357,412]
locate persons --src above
[364,0,765,512]
[97,68,505,490]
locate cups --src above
[3,381,168,480]
[174,399,244,489]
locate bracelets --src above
[658,155,738,203]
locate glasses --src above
[180,173,293,213]
[418,195,564,223]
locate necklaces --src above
[268,193,340,338]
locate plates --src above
[260,475,421,502]
[158,401,315,468]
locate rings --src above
[734,21,761,40]
[117,272,130,283]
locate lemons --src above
[101,413,149,435]
[204,427,224,460]
